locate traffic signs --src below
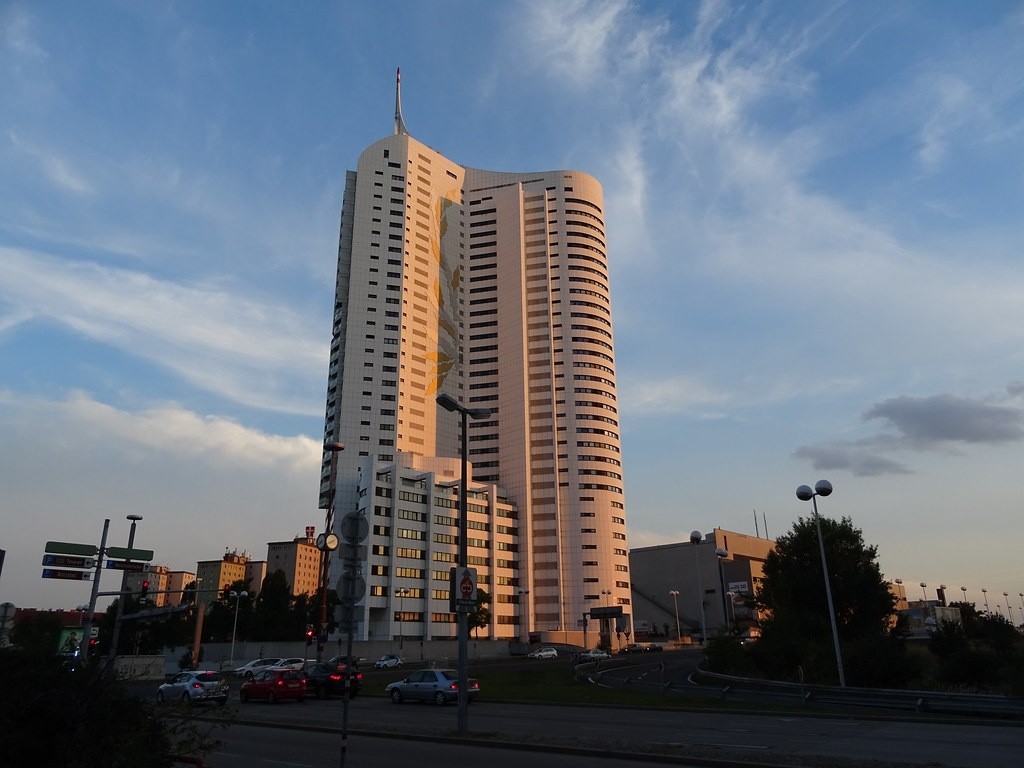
[41,554,95,581]
[106,560,150,573]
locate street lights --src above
[981,588,1024,627]
[895,578,904,608]
[961,586,967,601]
[105,515,143,677]
[229,591,249,669]
[727,592,735,623]
[394,586,411,661]
[315,442,349,663]
[796,479,845,689]
[920,583,929,607]
[670,591,682,642]
[435,393,492,735]
[518,589,530,647]
[690,530,710,648]
[715,547,729,633]
[602,589,611,607]
[76,604,90,627]
[940,585,947,607]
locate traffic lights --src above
[224,585,230,599]
[140,582,149,597]
[307,630,314,638]
[88,639,95,650]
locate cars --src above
[374,655,403,669]
[156,671,230,707]
[385,668,481,707]
[527,647,558,660]
[618,643,663,655]
[575,649,611,664]
